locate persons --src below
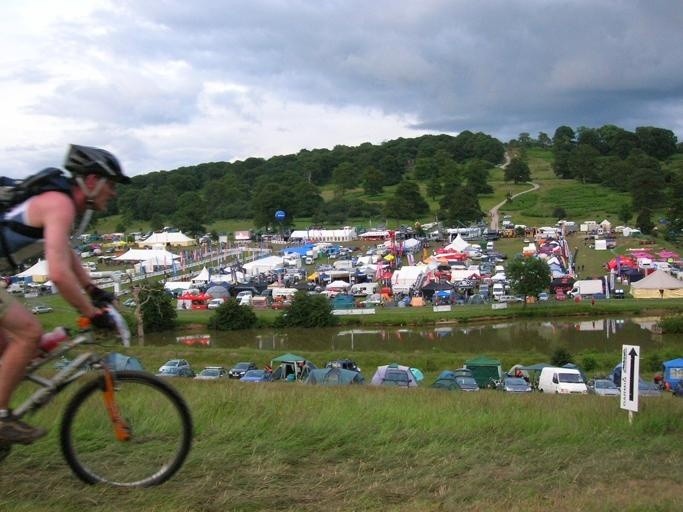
[0,144,128,445]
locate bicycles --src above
[0,288,192,493]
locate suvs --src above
[154,359,190,378]
[498,295,522,304]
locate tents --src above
[16,217,683,313]
[328,360,359,372]
[456,368,479,390]
[411,368,424,381]
[608,362,648,390]
[271,355,317,380]
[371,362,417,388]
[308,368,365,385]
[464,359,531,391]
[430,371,461,390]
[662,358,682,390]
[99,351,143,374]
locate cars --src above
[239,370,274,382]
[641,383,662,397]
[496,377,532,392]
[192,366,228,380]
[587,378,620,396]
[454,368,480,392]
[228,362,258,379]
[31,306,54,314]
[538,292,549,301]
[361,296,384,306]
[469,241,510,301]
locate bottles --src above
[32,325,69,358]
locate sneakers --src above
[0,408,46,444]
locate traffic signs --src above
[621,344,641,413]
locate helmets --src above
[65,144,131,184]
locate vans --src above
[538,367,587,395]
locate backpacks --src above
[1,167,79,267]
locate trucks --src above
[568,280,603,299]
[207,242,379,309]
